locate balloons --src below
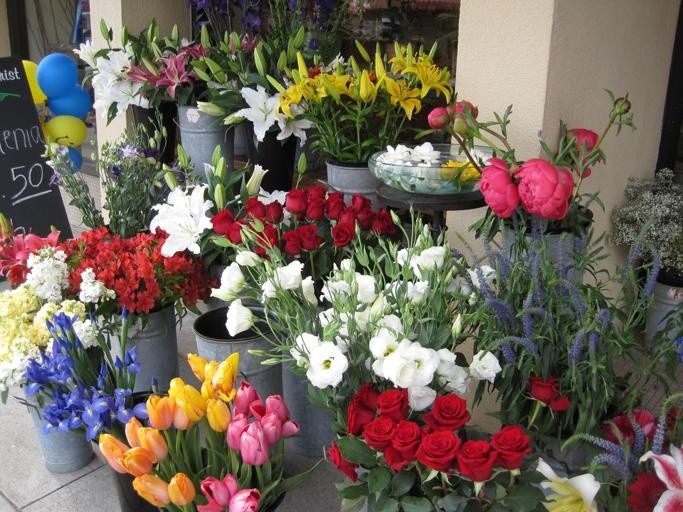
[52,143,84,177]
[40,113,89,152]
[35,53,78,100]
[41,81,91,121]
[20,60,48,109]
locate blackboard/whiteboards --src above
[0,55,74,244]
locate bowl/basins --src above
[367,143,503,195]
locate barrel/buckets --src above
[23,97,683,511]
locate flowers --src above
[1,0,683,512]
